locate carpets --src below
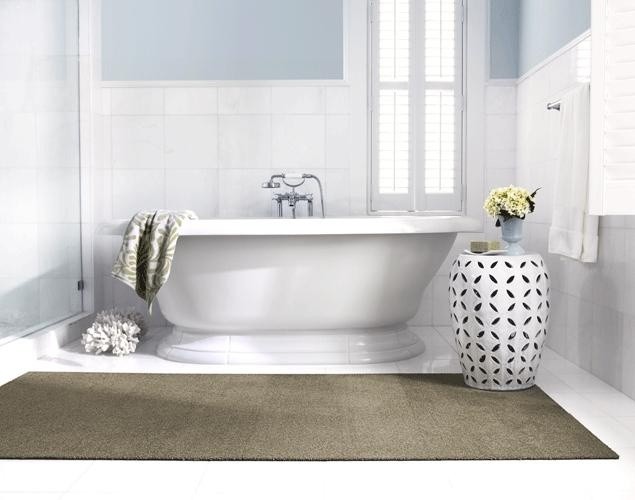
[0,371,621,461]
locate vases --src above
[495,214,527,255]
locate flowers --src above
[480,183,543,228]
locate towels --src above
[107,209,198,317]
[547,83,600,264]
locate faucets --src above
[272,191,313,217]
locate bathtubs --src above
[96,216,485,366]
